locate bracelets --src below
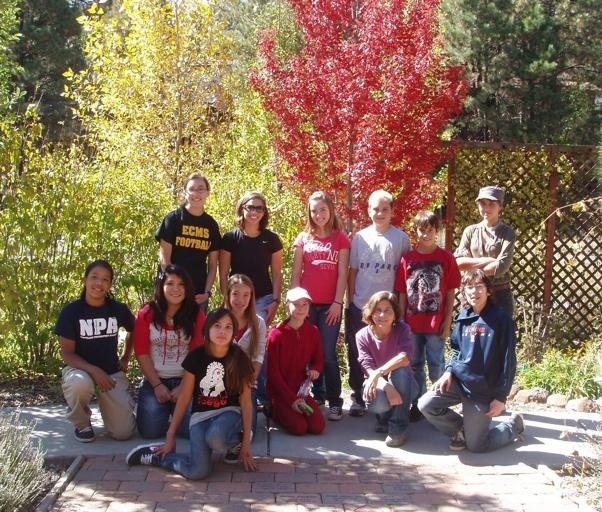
[154,383,163,389]
[334,301,343,307]
[205,290,212,297]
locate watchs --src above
[273,298,280,303]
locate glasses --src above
[185,187,207,193]
[463,284,486,291]
[242,204,265,213]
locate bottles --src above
[297,374,314,400]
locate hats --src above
[285,287,312,302]
[475,186,505,202]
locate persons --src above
[261,287,326,434]
[132,263,206,439]
[219,191,284,402]
[54,260,137,442]
[218,274,267,434]
[344,190,412,415]
[290,190,351,421]
[393,210,461,420]
[417,269,524,453]
[355,291,420,447]
[454,186,515,319]
[126,309,257,479]
[155,174,223,312]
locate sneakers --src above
[223,430,254,465]
[448,426,466,451]
[408,405,422,421]
[510,411,524,434]
[125,442,166,467]
[375,420,388,433]
[313,397,325,407]
[328,407,342,421]
[73,424,95,442]
[386,430,408,446]
[350,401,366,416]
